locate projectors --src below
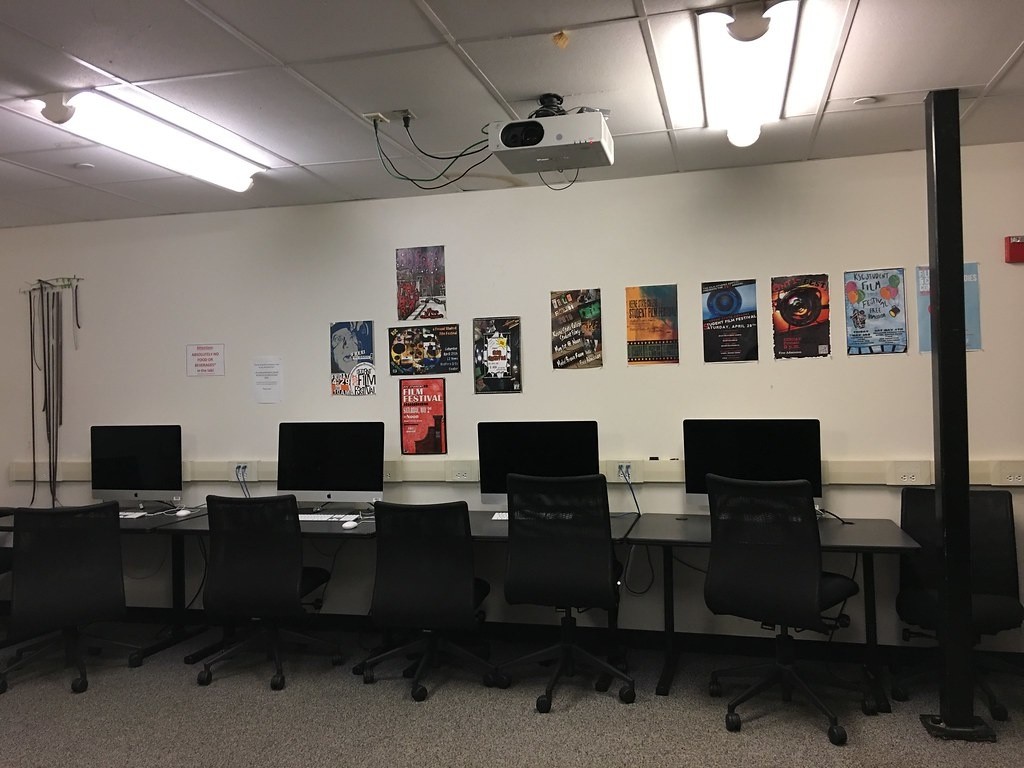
[488,113,616,174]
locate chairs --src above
[497,472,638,712]
[197,494,345,690]
[0,502,144,690]
[703,473,874,743]
[893,485,1020,721]
[354,501,514,697]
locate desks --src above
[352,508,637,691]
[156,512,375,665]
[0,506,207,669]
[627,512,922,702]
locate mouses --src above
[176,509,191,517]
[342,521,358,529]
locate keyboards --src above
[492,512,574,520]
[119,511,147,518]
[299,514,358,521]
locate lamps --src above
[693,0,807,149]
[23,87,269,194]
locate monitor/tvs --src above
[277,421,384,515]
[90,424,182,514]
[684,419,821,507]
[478,421,599,504]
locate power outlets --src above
[229,460,259,482]
[886,459,931,485]
[363,113,390,125]
[383,458,401,482]
[605,459,643,483]
[394,109,417,121]
[445,460,479,482]
[989,458,1024,485]
